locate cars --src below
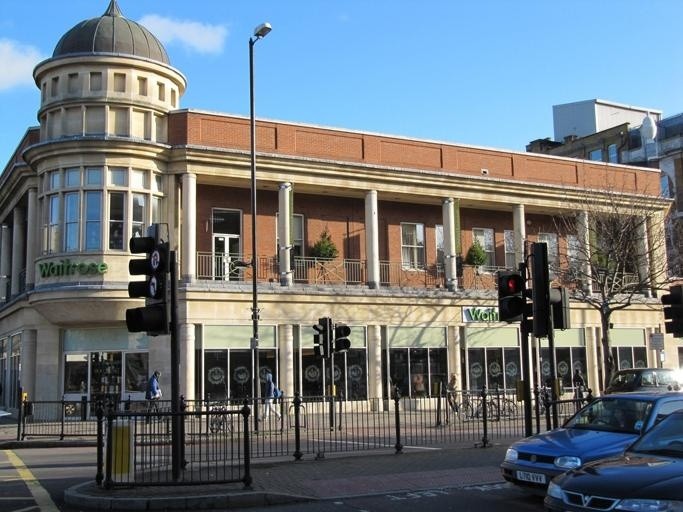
[541,408,683,511]
[500,390,683,499]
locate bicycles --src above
[456,390,565,423]
[209,399,233,434]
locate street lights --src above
[231,257,259,436]
[247,20,273,423]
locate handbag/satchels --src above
[274,388,282,399]
[150,390,162,399]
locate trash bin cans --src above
[104,420,135,487]
[368,399,379,411]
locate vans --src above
[603,366,683,413]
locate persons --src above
[445,371,459,415]
[413,373,425,397]
[572,369,587,407]
[257,372,281,422]
[145,370,163,426]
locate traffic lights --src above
[312,317,331,359]
[124,223,172,336]
[661,283,683,338]
[498,268,526,322]
[519,288,538,335]
[337,322,353,354]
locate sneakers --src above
[262,414,281,424]
[147,417,162,424]
